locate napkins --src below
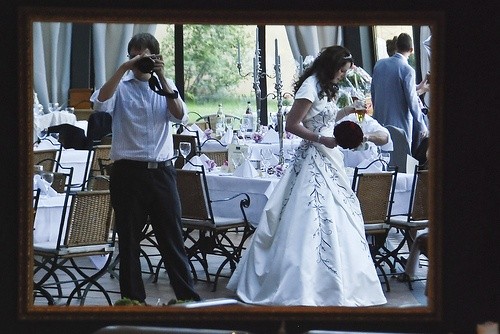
[191,123,204,140]
[180,126,196,136]
[181,155,207,175]
[232,159,259,177]
[219,126,233,145]
[261,128,279,144]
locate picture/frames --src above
[15,7,447,324]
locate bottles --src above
[231,130,239,145]
[213,116,249,143]
[243,101,254,132]
[216,104,225,124]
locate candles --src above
[300,55,303,75]
[275,39,279,83]
[238,44,240,64]
[253,28,259,83]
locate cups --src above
[354,100,366,125]
[65,107,74,114]
[36,127,48,140]
[50,133,59,141]
[240,146,252,160]
[48,102,59,113]
[33,164,44,178]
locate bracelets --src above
[316,134,322,143]
[342,108,347,117]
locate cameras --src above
[135,56,155,73]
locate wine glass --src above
[179,141,191,165]
[232,152,242,168]
[40,172,54,199]
[259,147,273,174]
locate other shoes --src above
[398,275,415,283]
[400,256,407,267]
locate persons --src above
[370,32,432,171]
[395,232,429,283]
[334,111,394,174]
[90,32,206,303]
[284,46,368,305]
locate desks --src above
[33,194,73,246]
[201,166,418,229]
[52,149,94,192]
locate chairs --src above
[33,100,429,306]
